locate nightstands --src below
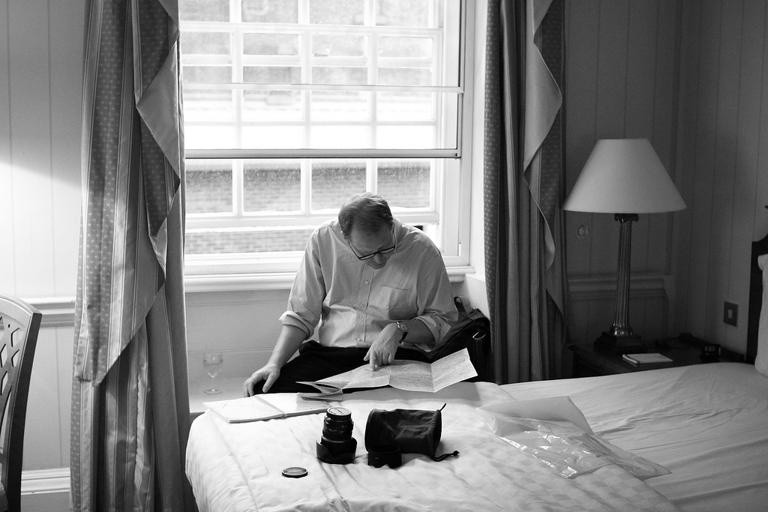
[565,339,730,375]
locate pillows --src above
[755,254,768,375]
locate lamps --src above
[563,138,688,346]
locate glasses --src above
[346,235,395,262]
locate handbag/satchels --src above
[428,296,496,376]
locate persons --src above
[242,191,459,400]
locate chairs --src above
[0,293,42,512]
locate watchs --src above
[394,320,409,344]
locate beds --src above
[185,360,768,512]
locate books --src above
[622,351,674,366]
[202,393,333,424]
